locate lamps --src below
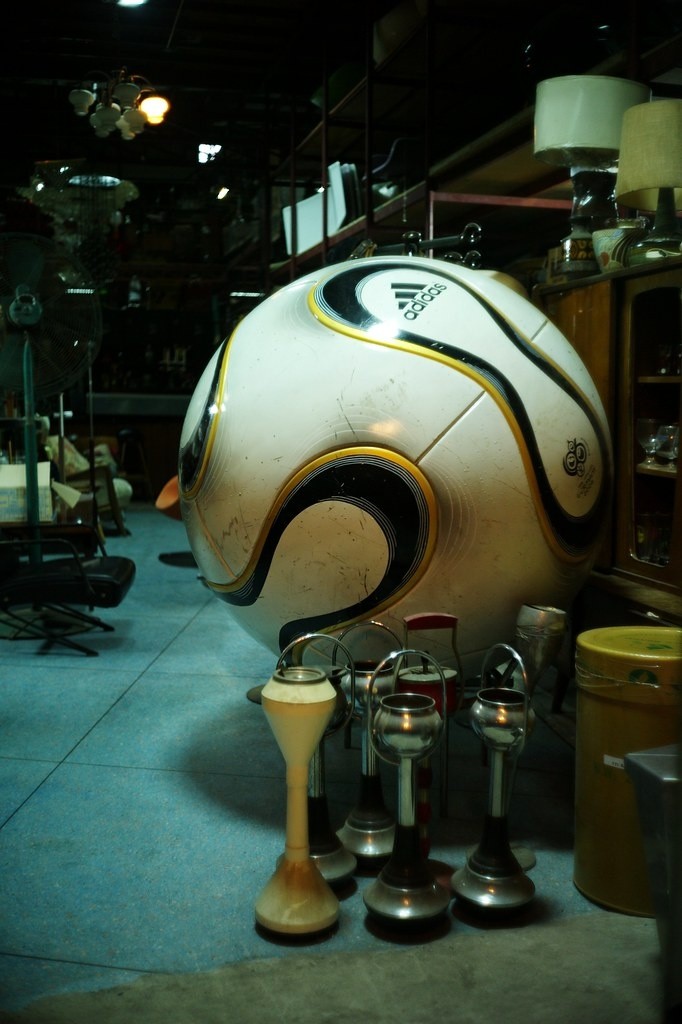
[69,61,179,142]
[529,73,656,276]
[609,98,680,268]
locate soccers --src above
[177,255,617,702]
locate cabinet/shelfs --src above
[610,272,681,586]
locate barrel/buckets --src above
[574,626,681,917]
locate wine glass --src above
[633,416,679,474]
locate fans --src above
[0,240,104,650]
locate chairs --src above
[0,522,135,657]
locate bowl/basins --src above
[591,229,648,273]
